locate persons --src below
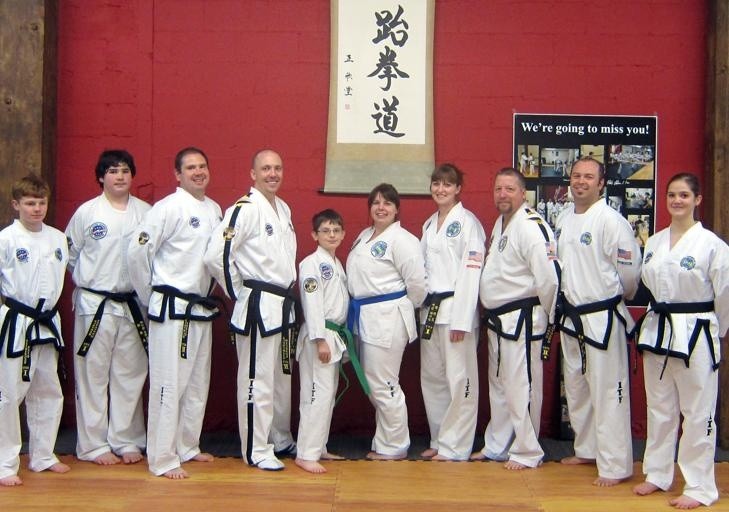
[520,152,536,175]
[556,156,643,489]
[630,193,642,209]
[345,182,429,462]
[0,175,71,487]
[642,192,653,210]
[537,197,546,221]
[294,207,351,475]
[632,172,729,510]
[634,219,650,248]
[126,145,223,480]
[546,197,574,226]
[64,149,154,467]
[469,166,564,472]
[202,148,300,471]
[419,161,487,462]
